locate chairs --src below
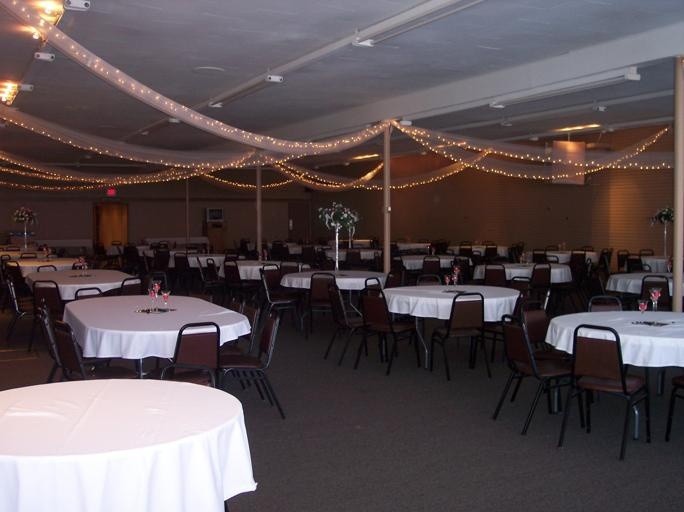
[492,245,682,462]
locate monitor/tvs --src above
[206,207,224,222]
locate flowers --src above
[646,203,674,230]
[12,204,39,238]
[314,200,363,235]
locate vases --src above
[663,223,667,260]
[334,228,339,277]
[23,230,27,251]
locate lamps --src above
[487,67,642,112]
[551,131,586,185]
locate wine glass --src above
[444,264,461,292]
[147,278,171,311]
[637,286,661,326]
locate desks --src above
[381,284,524,369]
[284,271,387,341]
[550,310,683,440]
[107,237,530,383]
[0,241,287,421]
[65,296,249,376]
[27,270,139,300]
